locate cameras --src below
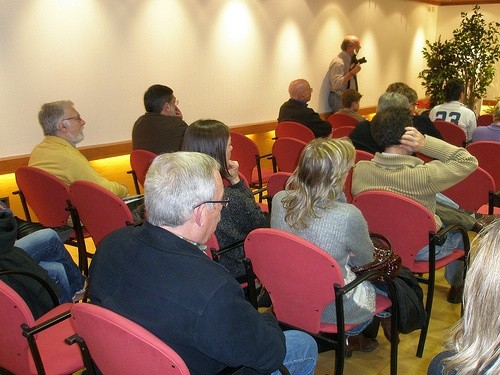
[351,55,367,66]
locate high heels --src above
[344,334,379,358]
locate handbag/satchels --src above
[353,233,400,281]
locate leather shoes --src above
[472,213,497,233]
[447,284,463,303]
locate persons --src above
[278,79,332,137]
[429,78,477,145]
[0,201,90,319]
[351,108,500,302]
[336,89,365,122]
[132,84,188,154]
[427,219,500,375]
[271,138,400,352]
[28,102,144,221]
[89,151,317,375]
[386,82,443,140]
[472,101,500,195]
[328,35,362,112]
[350,92,410,155]
[184,118,269,307]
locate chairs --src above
[0,113,500,375]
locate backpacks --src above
[391,265,428,335]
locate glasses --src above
[192,195,230,209]
[411,103,418,110]
[61,113,81,123]
[303,88,313,94]
